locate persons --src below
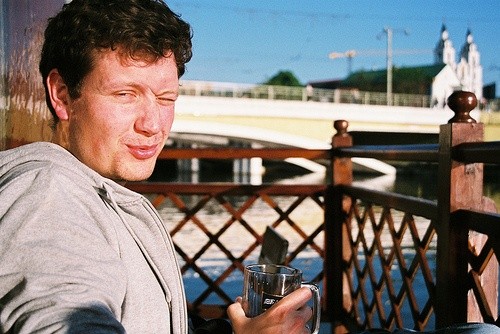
[430,97,499,113]
[304,82,362,104]
[0,0,315,334]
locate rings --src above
[305,325,310,330]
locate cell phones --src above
[250,226,289,295]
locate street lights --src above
[377,27,408,106]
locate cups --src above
[232,263,321,334]
